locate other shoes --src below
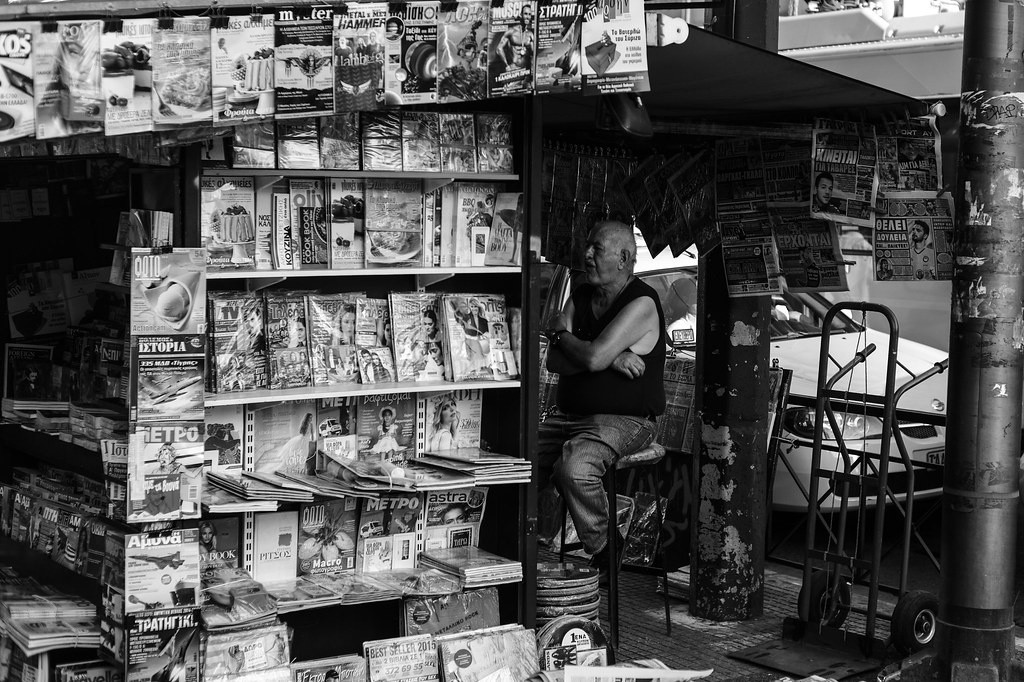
[588,529,626,578]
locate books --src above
[0,0,655,682]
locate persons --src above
[300,351,309,364]
[294,363,302,376]
[812,172,842,216]
[384,320,392,348]
[336,356,345,371]
[17,366,46,401]
[361,350,377,382]
[428,341,445,379]
[280,412,314,471]
[424,310,440,341]
[410,341,428,381]
[237,353,256,390]
[454,298,489,359]
[295,316,307,348]
[370,406,407,463]
[286,364,294,376]
[233,373,245,390]
[151,441,203,477]
[221,357,246,391]
[346,360,354,374]
[333,303,355,345]
[496,4,535,71]
[243,307,265,350]
[540,220,666,579]
[437,36,487,97]
[429,395,461,451]
[290,351,297,363]
[441,504,467,525]
[371,352,391,383]
[200,523,237,570]
[898,141,921,163]
[877,259,893,281]
[37,23,103,126]
[909,220,936,280]
[327,347,336,373]
[279,351,289,376]
[666,278,812,345]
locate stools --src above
[559,440,670,651]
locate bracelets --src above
[546,328,568,346]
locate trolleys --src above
[725,299,949,682]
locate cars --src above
[537,223,951,516]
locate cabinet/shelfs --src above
[1,110,526,682]
